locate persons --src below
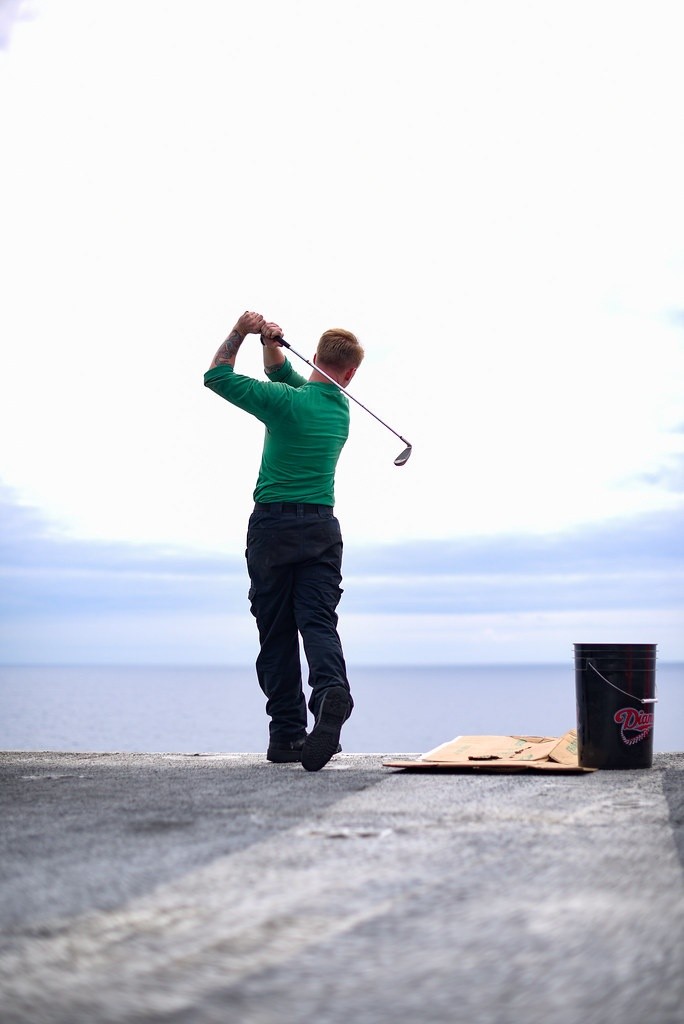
[203,309,364,771]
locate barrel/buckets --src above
[570,643,660,770]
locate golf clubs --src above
[277,336,412,466]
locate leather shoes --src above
[301,687,351,771]
[267,736,343,763]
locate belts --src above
[253,502,333,515]
